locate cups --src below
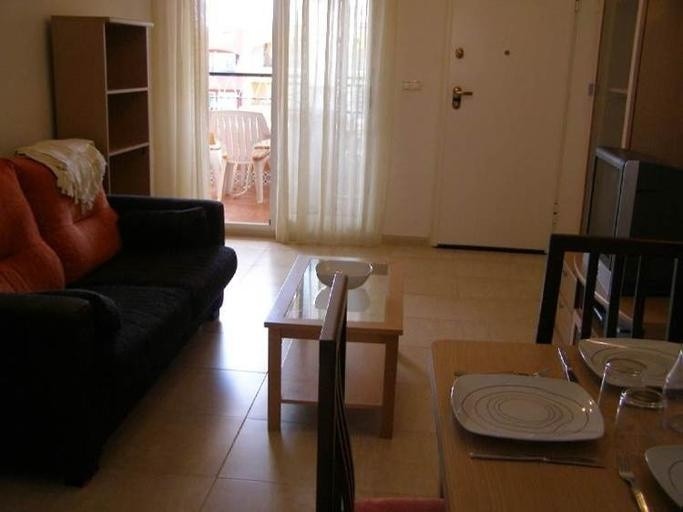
[611,387,669,464]
[595,357,650,434]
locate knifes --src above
[556,346,579,384]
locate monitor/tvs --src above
[583,146,683,296]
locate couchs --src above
[1,136,236,488]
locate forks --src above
[615,453,651,511]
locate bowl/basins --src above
[316,259,374,289]
[313,286,371,313]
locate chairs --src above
[210,111,270,204]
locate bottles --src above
[660,347,682,435]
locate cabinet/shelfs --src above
[580,0,683,231]
[555,252,673,347]
[48,16,155,198]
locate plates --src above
[643,445,683,510]
[449,372,605,444]
[579,337,682,390]
